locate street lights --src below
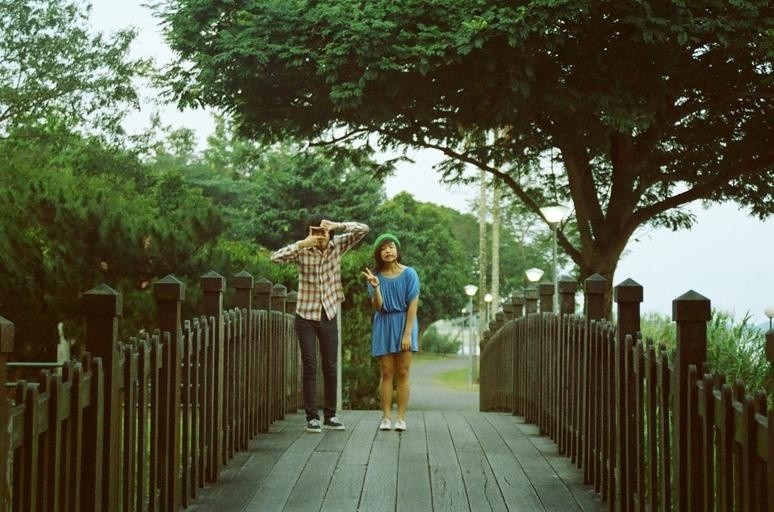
[465,284,478,386]
[539,202,569,314]
[461,308,467,357]
[483,293,493,329]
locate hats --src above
[373,234,401,255]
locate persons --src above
[268,214,370,432]
[361,232,421,432]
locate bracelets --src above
[373,283,380,289]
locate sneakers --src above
[323,418,345,430]
[307,418,322,432]
[380,417,392,430]
[394,418,406,431]
